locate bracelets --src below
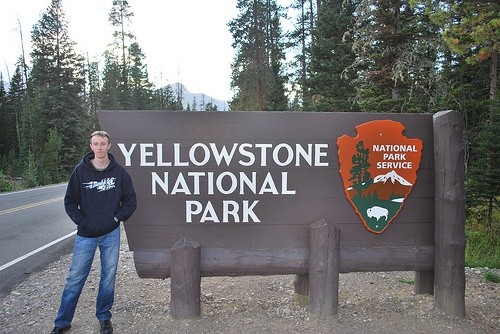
[114,216,118,224]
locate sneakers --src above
[50,325,71,334]
[99,319,113,334]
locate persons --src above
[49,129,138,333]
